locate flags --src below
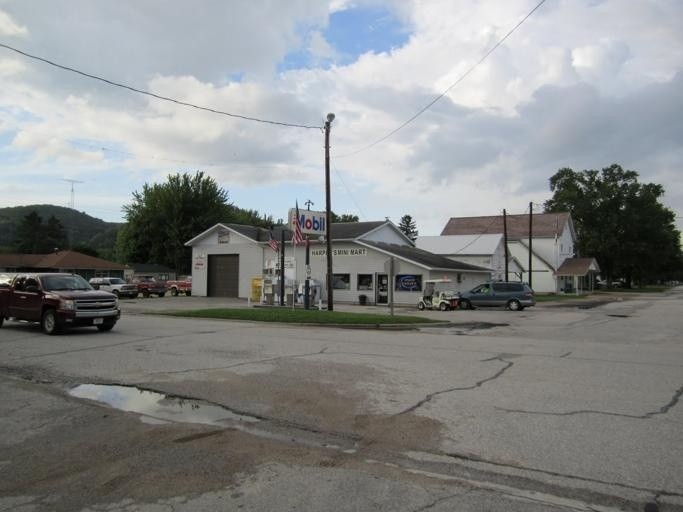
[266,229,280,252]
[291,204,302,247]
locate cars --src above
[597,279,628,288]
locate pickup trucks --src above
[1,271,121,338]
[165,275,193,297]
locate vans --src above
[457,280,537,311]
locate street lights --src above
[323,112,337,312]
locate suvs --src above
[129,273,168,300]
[89,276,137,299]
